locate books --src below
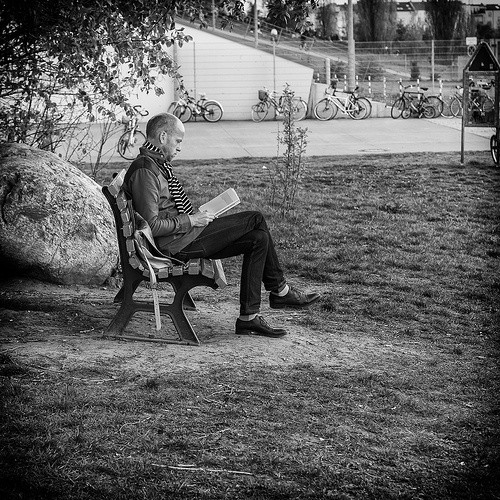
[198,187,240,218]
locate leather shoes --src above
[269,285,320,309]
[235,315,287,338]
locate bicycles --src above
[166,80,224,124]
[117,104,148,161]
[390,82,495,119]
[270,35,279,45]
[299,42,310,51]
[313,79,373,121]
[251,86,309,123]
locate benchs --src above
[100,169,228,346]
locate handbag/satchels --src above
[127,200,186,330]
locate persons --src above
[176,1,405,57]
[123,113,322,339]
